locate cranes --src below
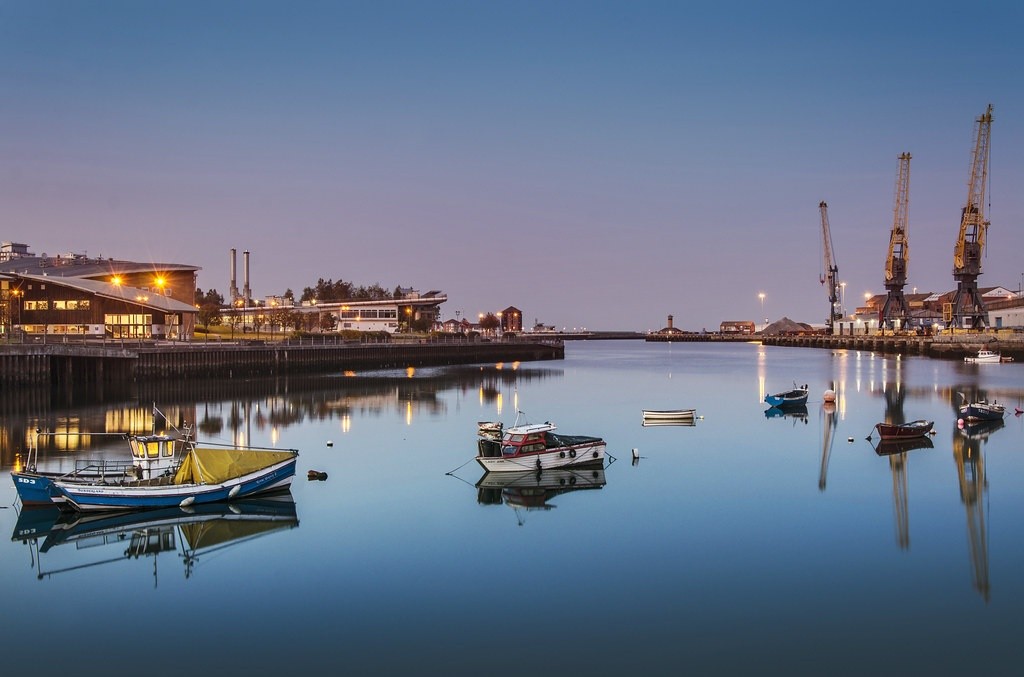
[953,103,995,283]
[814,201,847,303]
[882,151,912,291]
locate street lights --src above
[841,283,846,311]
[760,293,766,331]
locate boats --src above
[10,432,298,513]
[474,421,606,470]
[877,420,935,438]
[11,501,300,558]
[642,409,697,419]
[960,403,1005,421]
[475,470,607,489]
[965,351,1013,363]
[763,387,809,408]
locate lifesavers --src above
[569,477,576,484]
[570,449,576,457]
